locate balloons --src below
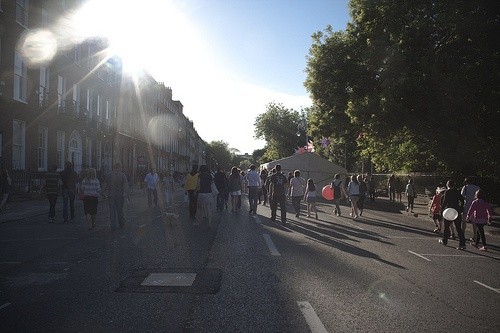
[322,185,334,200]
[443,208,458,220]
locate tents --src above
[259,152,347,198]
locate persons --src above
[229,167,243,214]
[0,156,14,209]
[439,180,466,250]
[405,179,417,213]
[163,163,295,229]
[461,177,492,251]
[389,176,403,201]
[430,183,460,239]
[43,161,79,224]
[269,164,287,223]
[80,168,103,231]
[144,167,160,208]
[109,162,125,230]
[304,178,318,219]
[246,164,261,214]
[286,170,306,217]
[330,172,377,218]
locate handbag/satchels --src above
[210,183,219,196]
[414,192,417,198]
[78,192,84,200]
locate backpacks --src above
[332,181,341,199]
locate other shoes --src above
[282,220,286,225]
[479,246,487,251]
[93,223,96,226]
[456,247,467,250]
[434,227,439,232]
[407,208,408,212]
[411,211,414,213]
[271,216,275,221]
[49,219,55,222]
[89,225,92,230]
[439,239,447,245]
[469,241,478,247]
